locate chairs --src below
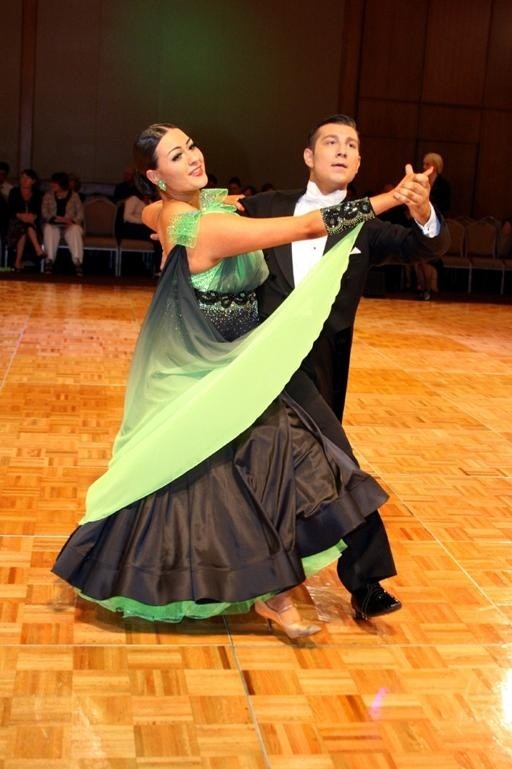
[0,196,159,277]
[369,217,512,294]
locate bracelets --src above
[319,198,377,238]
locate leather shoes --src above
[351,581,402,620]
[15,252,91,274]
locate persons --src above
[112,168,155,206]
[148,117,451,621]
[53,123,435,640]
[207,172,274,196]
[0,161,87,277]
[349,153,451,303]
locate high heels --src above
[255,590,323,640]
